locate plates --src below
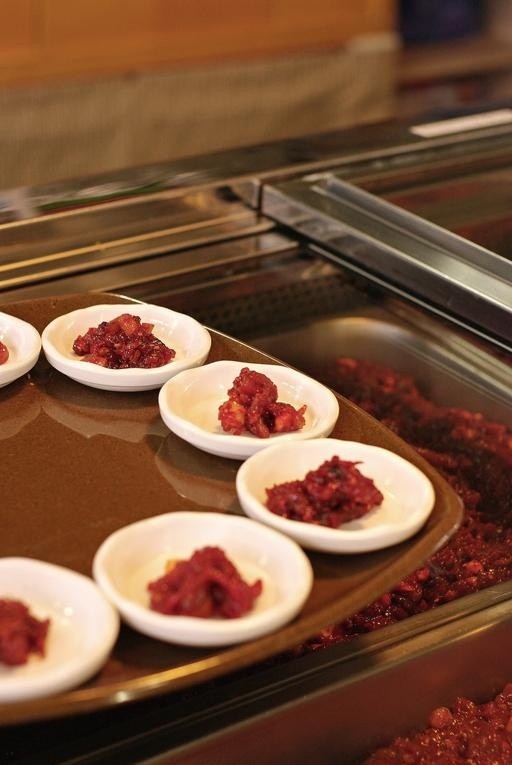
[42,305,213,391]
[236,439,435,553]
[94,512,312,645]
[3,556,120,702]
[159,360,340,460]
[2,308,42,389]
